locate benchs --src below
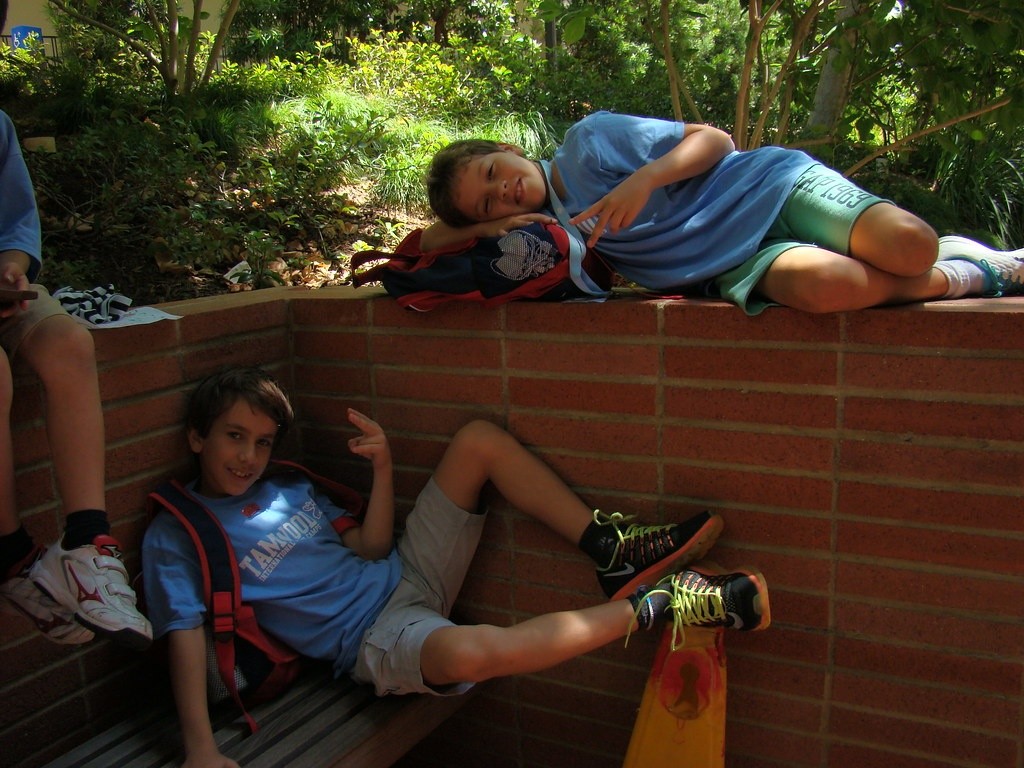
[0,544,485,768]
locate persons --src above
[141,368,771,768]
[0,0,153,648]
[419,111,1024,316]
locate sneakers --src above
[29,532,154,647]
[936,236,1024,295]
[0,545,96,645]
[623,558,772,653]
[593,508,725,604]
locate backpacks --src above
[353,222,590,311]
